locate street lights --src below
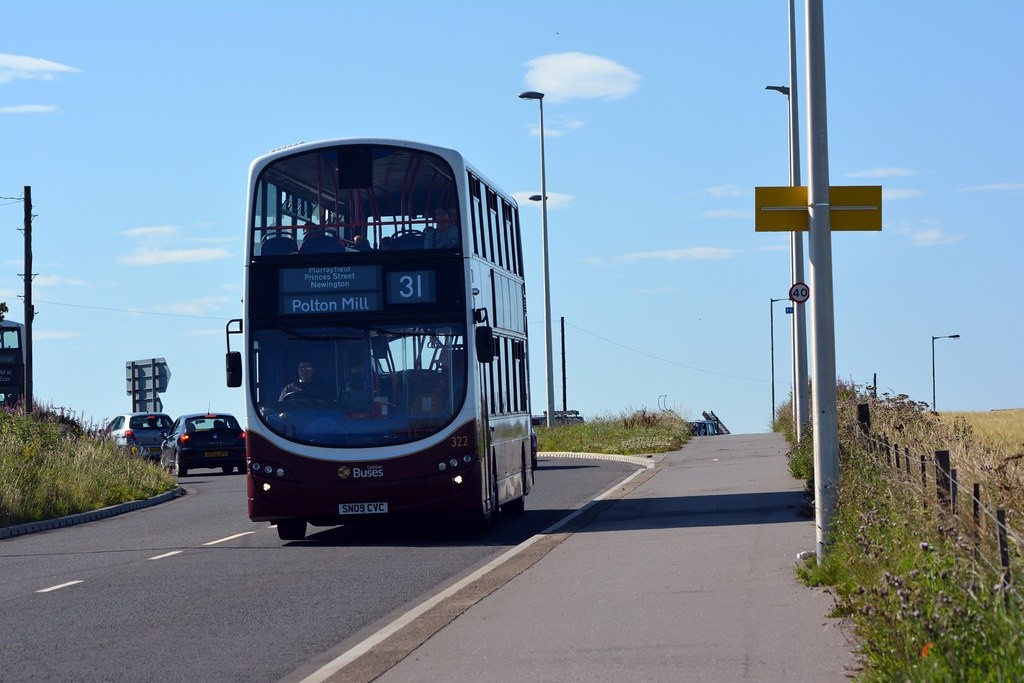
[932,334,961,411]
[518,90,556,429]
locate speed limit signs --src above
[790,281,810,303]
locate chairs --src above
[261,231,299,256]
[389,230,425,250]
[214,421,226,430]
[298,230,345,255]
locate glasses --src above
[298,367,314,372]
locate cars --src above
[99,411,173,461]
[161,413,248,477]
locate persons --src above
[297,220,319,250]
[353,234,362,242]
[422,206,461,250]
[276,358,325,401]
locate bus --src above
[223,136,537,542]
